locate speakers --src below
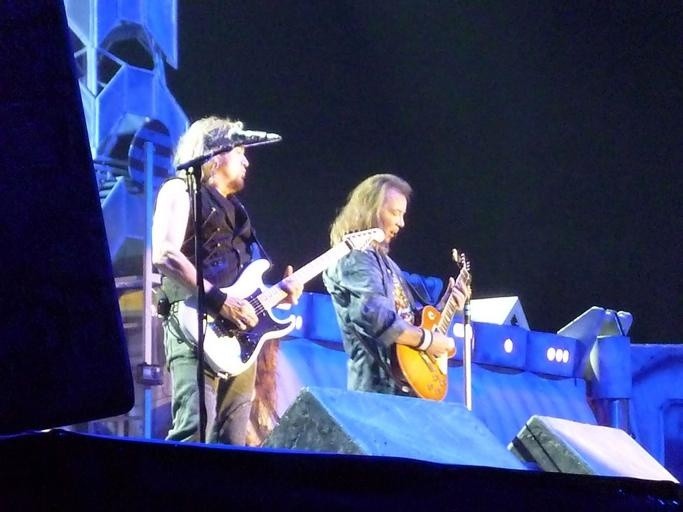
[0,0,136,438]
[506,415,681,484]
[257,388,530,471]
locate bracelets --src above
[203,282,226,312]
[416,326,434,353]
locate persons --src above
[321,173,474,401]
[148,115,305,448]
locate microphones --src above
[228,126,279,141]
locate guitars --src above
[166,226,384,381]
[391,248,473,400]
